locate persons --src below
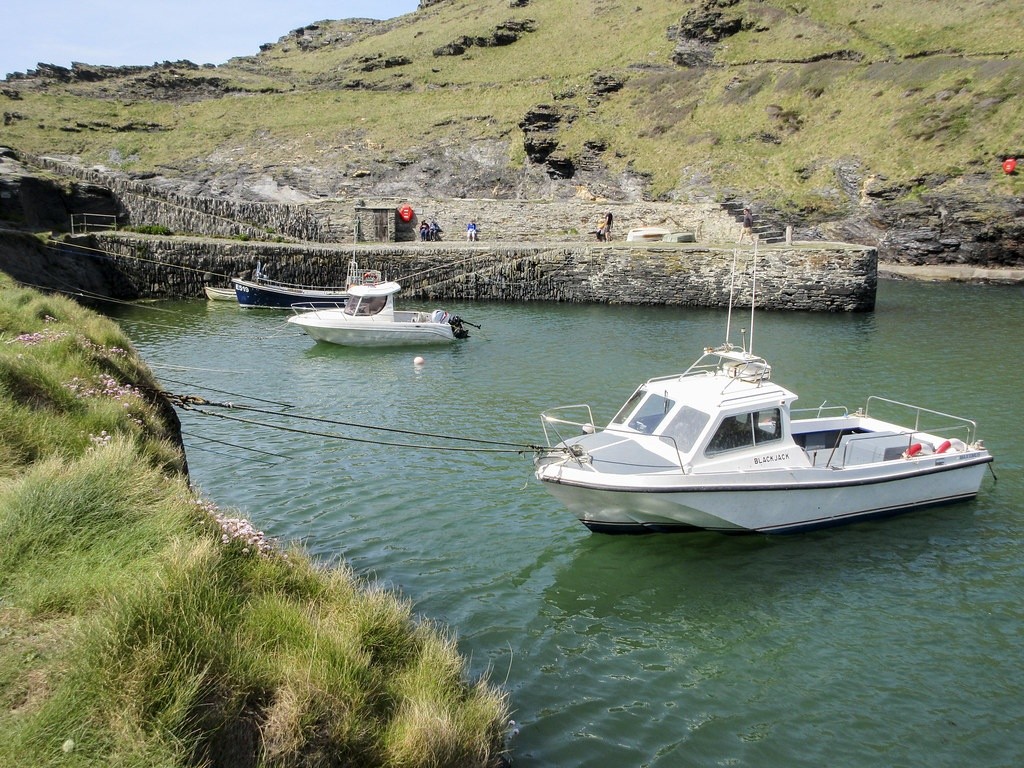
[605,213,613,242]
[596,220,605,242]
[429,220,439,242]
[419,220,430,242]
[467,219,476,242]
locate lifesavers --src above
[901,437,966,460]
[361,272,378,286]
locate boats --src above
[285,269,483,348]
[534,247,995,545]
[234,264,354,310]
[205,285,238,302]
